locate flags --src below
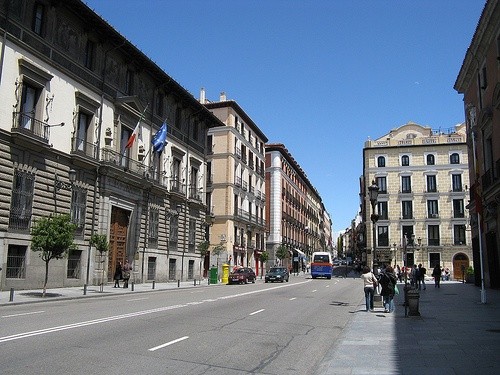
[151,119,168,153]
[125,119,141,148]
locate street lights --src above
[367,179,384,303]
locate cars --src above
[265,267,289,283]
[228,267,256,285]
[334,257,353,266]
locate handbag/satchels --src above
[394,284,400,295]
[376,283,382,295]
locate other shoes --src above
[383,309,393,313]
[418,288,421,290]
[435,285,438,288]
[438,285,440,288]
[366,309,373,312]
[423,287,426,290]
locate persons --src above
[114,259,130,288]
[411,264,426,290]
[433,264,441,288]
[441,268,451,280]
[378,263,400,312]
[361,266,377,312]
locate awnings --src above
[292,247,305,258]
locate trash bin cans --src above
[209,265,218,284]
[221,263,229,283]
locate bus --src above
[311,251,333,280]
